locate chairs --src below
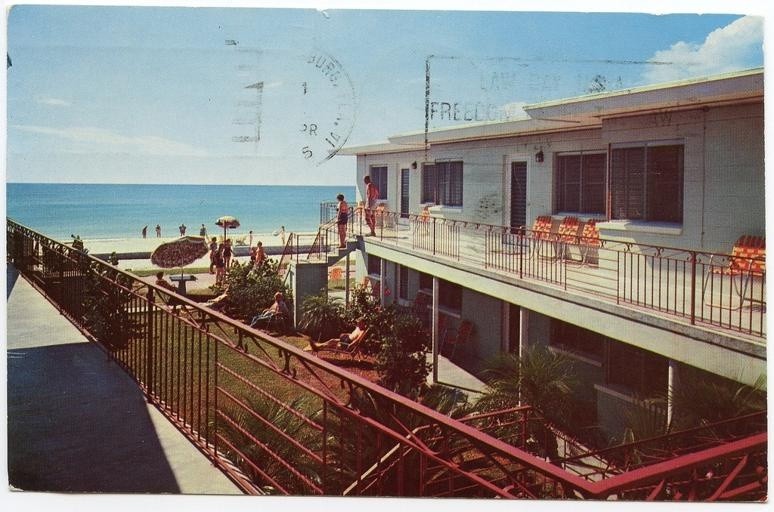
[513,215,606,266]
[355,276,380,301]
[374,202,384,217]
[440,322,474,361]
[303,327,372,361]
[355,201,365,215]
[702,235,765,310]
[414,206,432,234]
[261,301,293,332]
[417,315,447,351]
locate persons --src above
[156,223,161,238]
[309,315,366,352]
[278,225,286,247]
[154,271,188,313]
[362,175,380,237]
[208,236,217,274]
[199,223,206,240]
[222,239,236,271]
[212,242,225,286]
[178,223,187,237]
[248,291,288,330]
[249,230,253,246]
[334,192,349,249]
[255,240,265,264]
[249,247,257,261]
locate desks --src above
[169,275,196,295]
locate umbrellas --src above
[150,235,209,285]
